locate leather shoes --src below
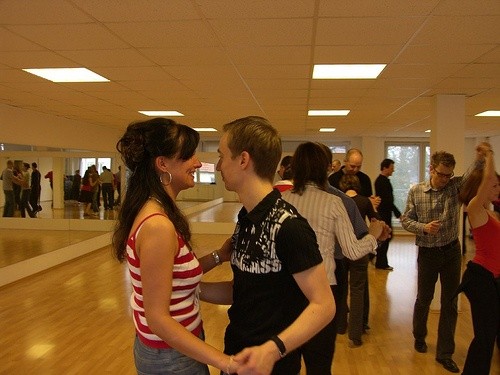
[435,358,460,373]
[415,338,427,353]
[383,265,393,270]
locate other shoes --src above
[350,337,363,345]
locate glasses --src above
[434,168,454,178]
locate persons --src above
[335,173,382,345]
[195,115,336,375]
[332,159,341,172]
[271,163,295,193]
[374,158,404,270]
[281,141,385,375]
[273,155,292,185]
[297,142,392,275]
[112,116,249,375]
[461,139,500,375]
[0,159,123,218]
[401,141,493,373]
[328,148,383,330]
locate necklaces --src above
[148,196,162,206]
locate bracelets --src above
[270,335,287,360]
[227,355,235,375]
[212,250,221,265]
[485,149,495,157]
